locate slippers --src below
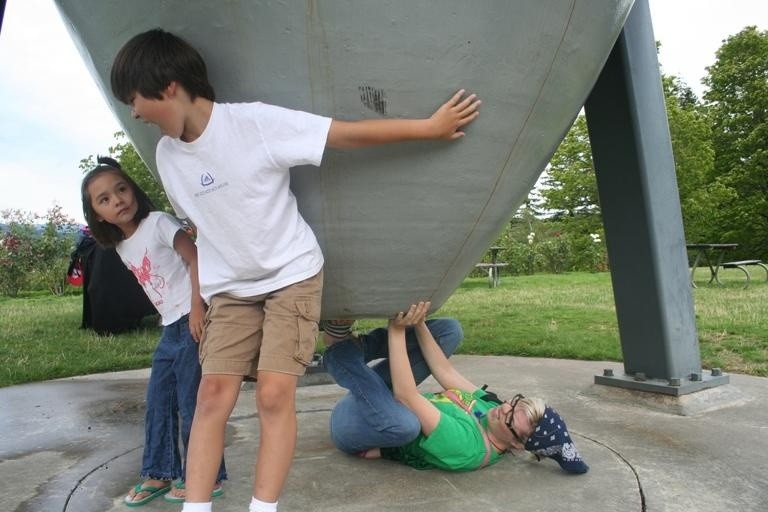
[123,480,223,506]
[318,317,356,340]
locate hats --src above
[524,406,593,475]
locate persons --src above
[110,27,480,512]
[79,154,228,508]
[314,300,571,475]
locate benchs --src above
[721,260,768,289]
[476,263,508,288]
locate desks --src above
[687,244,738,289]
[482,247,506,264]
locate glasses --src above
[504,393,543,464]
